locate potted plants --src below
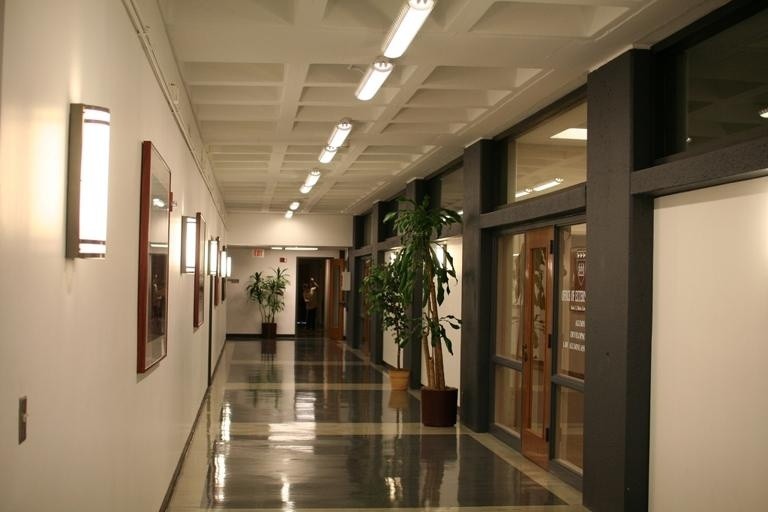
[358,189,460,429]
[242,268,292,340]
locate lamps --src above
[349,1,437,102]
[285,118,351,221]
[65,101,110,261]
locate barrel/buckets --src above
[387,391,410,410]
[390,369,409,391]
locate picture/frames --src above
[133,136,229,375]
